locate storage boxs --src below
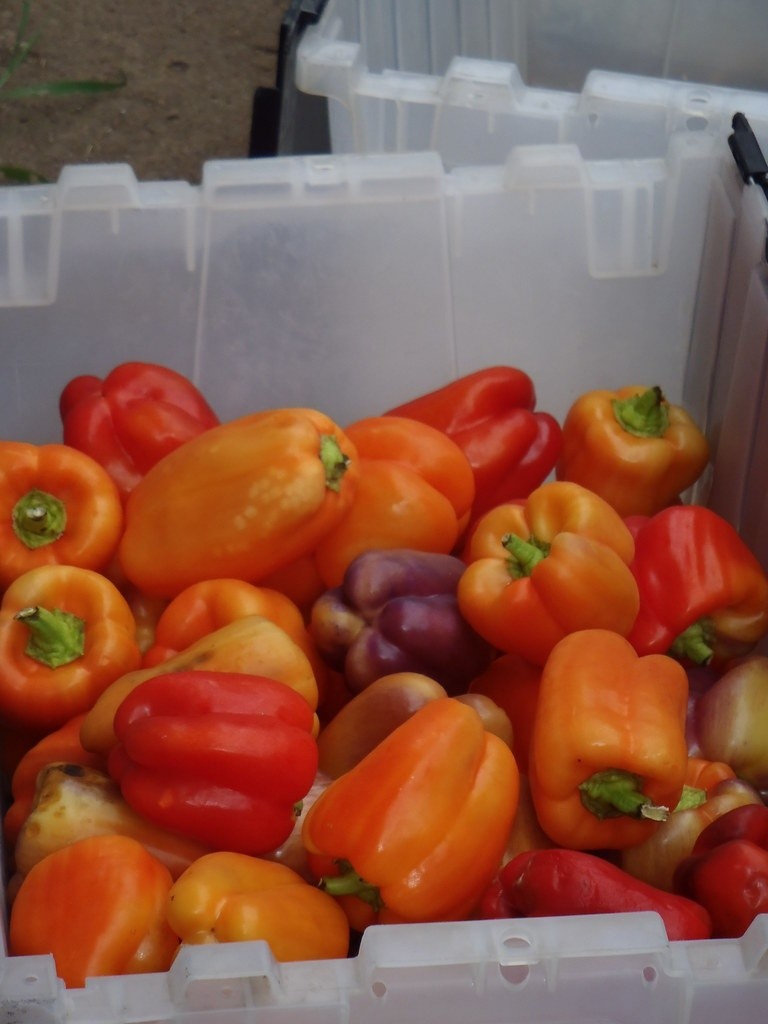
[292,1,768,162]
[1,137,765,1021]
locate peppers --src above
[0,360,768,985]
